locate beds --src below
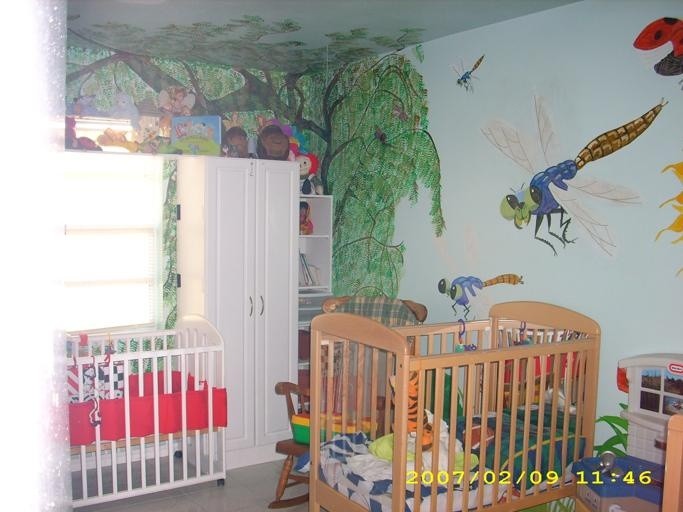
[66,312,227,508]
[306,300,602,511]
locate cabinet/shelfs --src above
[297,192,336,414]
[173,154,301,473]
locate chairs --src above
[266,292,428,511]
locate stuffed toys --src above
[63,114,318,234]
[390,369,437,452]
